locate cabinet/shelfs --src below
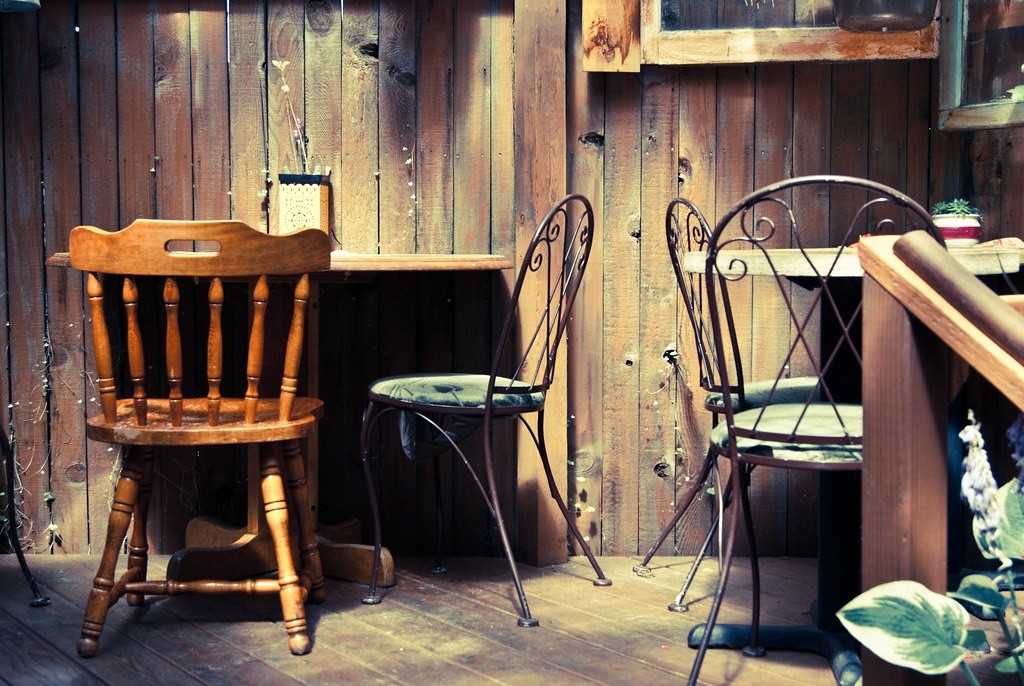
[580,0,938,72]
[861,234,1021,685]
[937,0,1024,128]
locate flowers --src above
[952,409,1011,575]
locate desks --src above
[677,245,1022,686]
[47,254,511,586]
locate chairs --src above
[360,196,610,628]
[691,177,947,686]
[69,219,330,655]
[636,197,821,609]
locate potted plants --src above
[933,200,983,249]
[271,61,333,236]
